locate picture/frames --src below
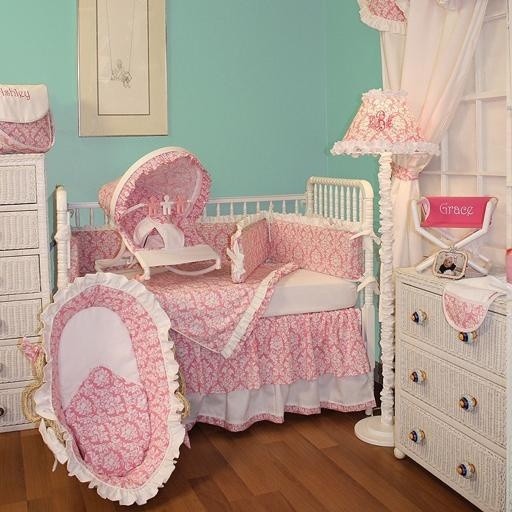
[433,249,468,279]
[76,0,168,137]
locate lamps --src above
[334,89,442,447]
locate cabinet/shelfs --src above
[0,152,51,432]
[395,261,511,508]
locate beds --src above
[54,176,375,416]
